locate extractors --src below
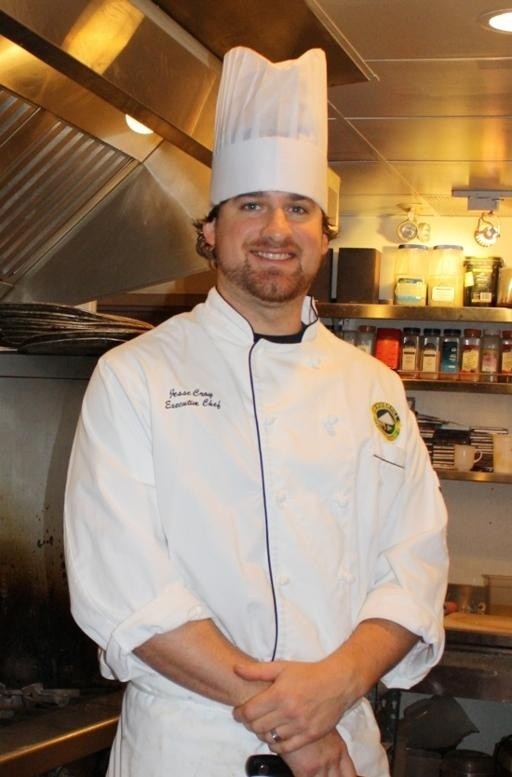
[0,2,387,323]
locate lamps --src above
[395,200,432,244]
[448,183,512,247]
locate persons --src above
[61,46,450,775]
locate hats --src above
[208,45,329,216]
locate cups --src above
[453,445,482,471]
[491,433,512,475]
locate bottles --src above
[324,321,512,382]
[393,241,505,306]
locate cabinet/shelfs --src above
[93,288,512,488]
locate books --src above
[413,409,508,472]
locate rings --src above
[269,726,286,743]
